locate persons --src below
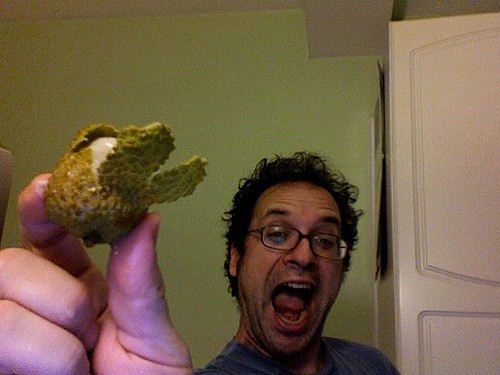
[1,147,401,374]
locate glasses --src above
[245,222,349,261]
